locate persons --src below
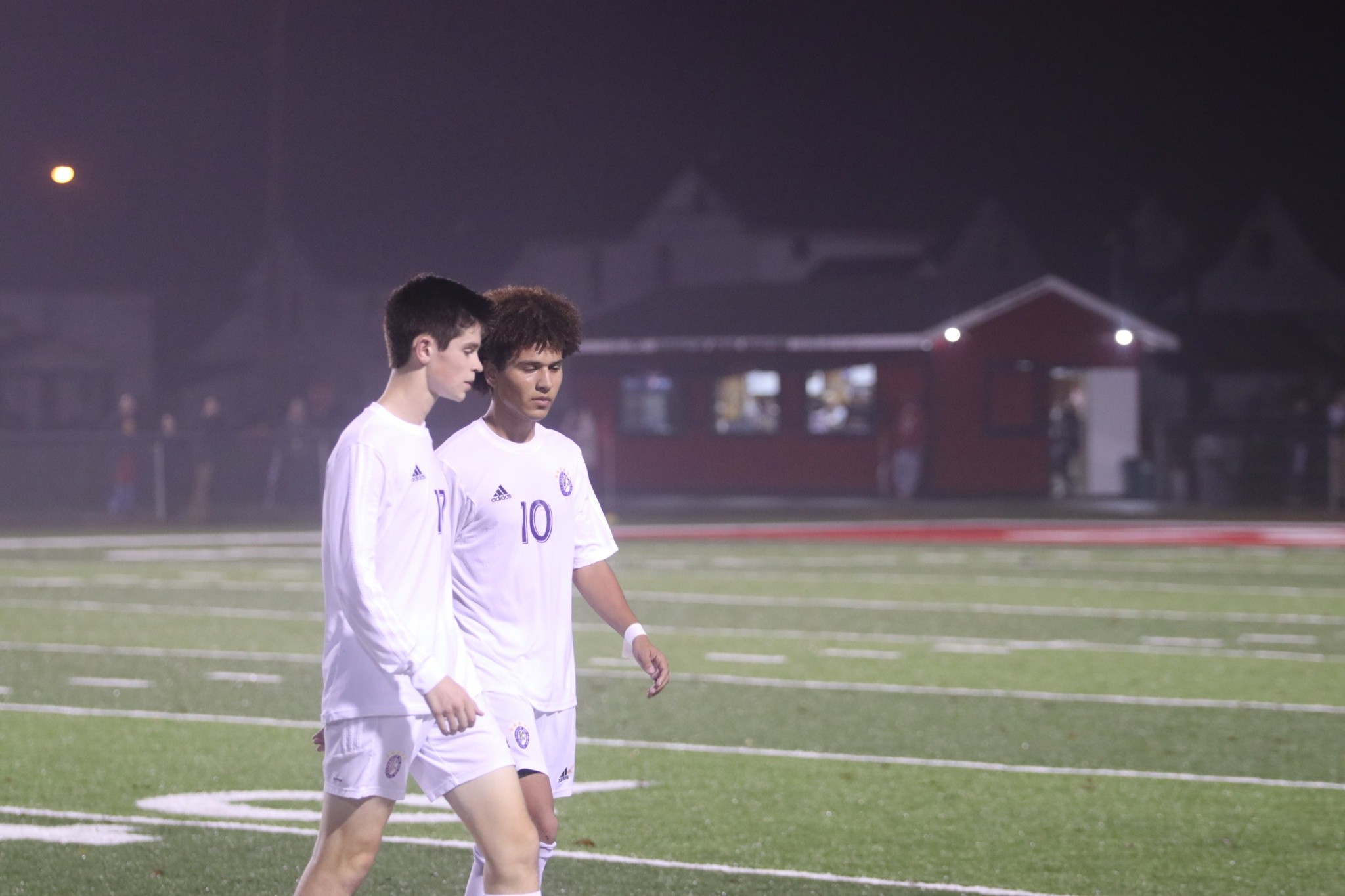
[186,389,224,515]
[273,394,321,504]
[98,392,143,514]
[1046,396,1083,501]
[289,275,546,896]
[806,385,852,437]
[433,288,670,896]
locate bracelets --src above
[621,623,648,658]
